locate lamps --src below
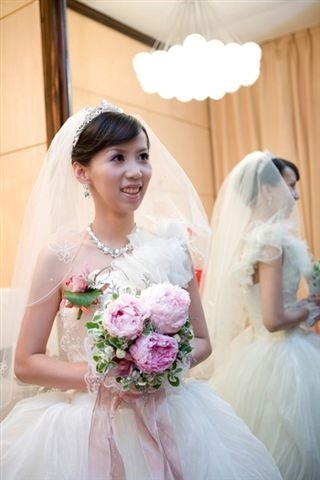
[133,1,262,103]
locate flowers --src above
[308,259,320,307]
[63,264,129,320]
[86,273,194,392]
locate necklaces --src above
[87,221,136,258]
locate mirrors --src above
[38,0,320,480]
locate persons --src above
[1,112,285,479]
[201,156,318,479]
